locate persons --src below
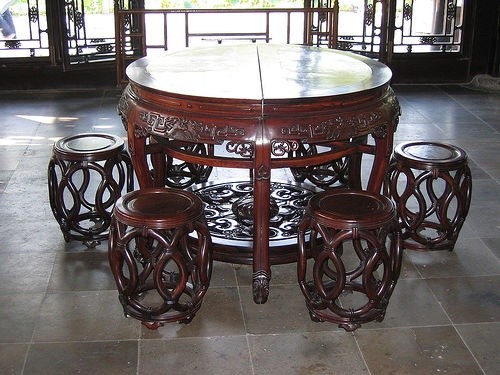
[0,0,17,39]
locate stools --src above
[108,187,214,330]
[288,134,368,190]
[151,138,214,189]
[48,133,133,249]
[297,189,403,332]
[384,141,473,251]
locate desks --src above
[116,43,402,305]
